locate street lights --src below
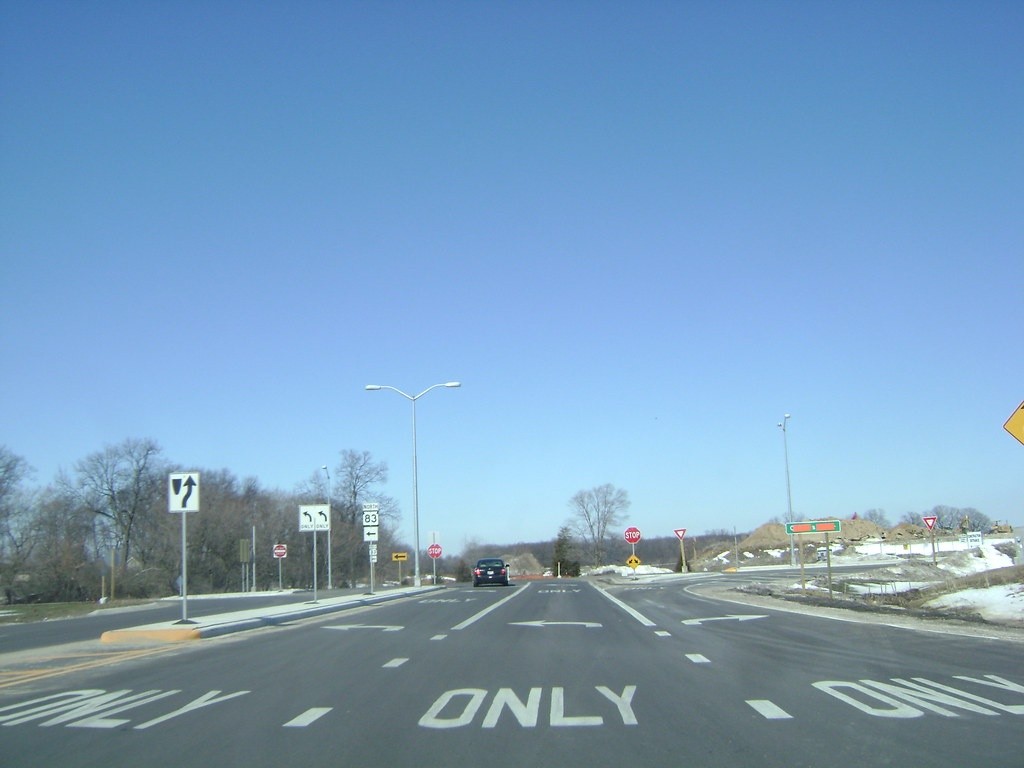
[777,413,796,566]
[322,466,334,590]
[366,382,460,588]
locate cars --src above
[471,558,510,587]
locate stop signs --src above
[624,526,641,544]
[427,544,442,559]
[273,544,287,558]
